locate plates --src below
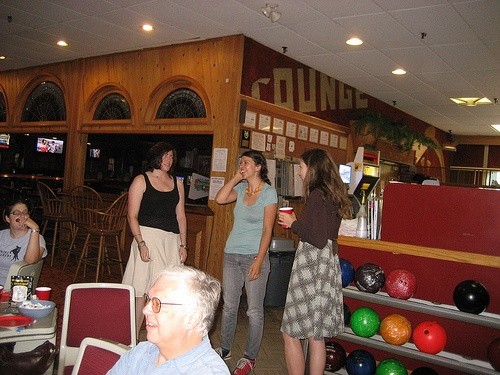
[0,316,33,329]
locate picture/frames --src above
[197,156,210,174]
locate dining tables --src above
[0,308,57,375]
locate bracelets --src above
[133,233,141,236]
[31,230,39,232]
[180,245,189,251]
[138,240,146,251]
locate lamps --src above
[261,4,282,24]
[443,130,456,150]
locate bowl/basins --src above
[16,299,56,318]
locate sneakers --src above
[215,347,231,360]
[232,357,257,375]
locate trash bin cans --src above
[263,237,296,306]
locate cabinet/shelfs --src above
[303,235,500,375]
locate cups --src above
[0,285,4,298]
[279,207,294,228]
[35,287,52,301]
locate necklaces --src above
[246,179,262,199]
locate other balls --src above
[324,303,447,375]
[338,257,356,288]
[355,261,385,294]
[487,337,500,372]
[384,268,416,300]
[453,280,490,315]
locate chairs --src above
[3,259,43,294]
[70,336,130,375]
[58,283,136,375]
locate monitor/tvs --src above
[36,137,65,155]
[89,148,101,158]
[0,133,10,148]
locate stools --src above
[73,192,129,283]
[34,181,80,267]
[63,186,114,278]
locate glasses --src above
[143,293,184,313]
[8,210,28,216]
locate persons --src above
[278,149,353,375]
[216,150,278,375]
[0,199,47,353]
[122,143,186,346]
[105,264,231,375]
